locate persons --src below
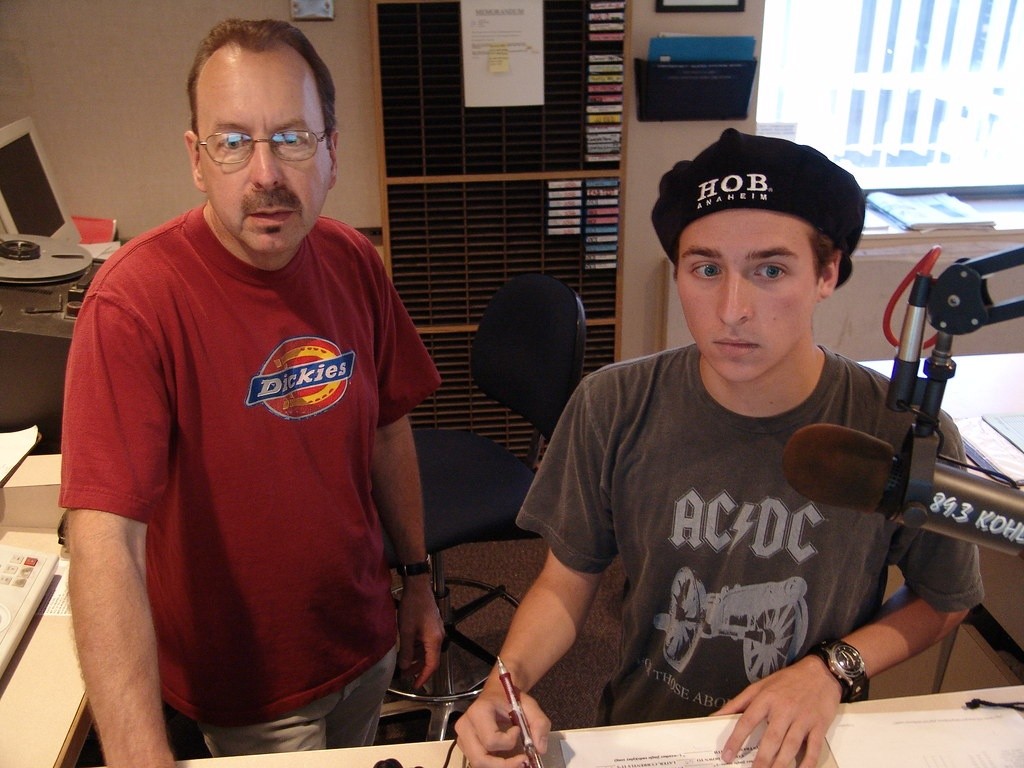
[61,18,446,768]
[455,129,984,768]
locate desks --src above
[0,352,1024,768]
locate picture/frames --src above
[656,0,746,13]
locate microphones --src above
[780,423,1024,560]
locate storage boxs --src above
[0,454,67,528]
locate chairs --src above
[366,273,588,743]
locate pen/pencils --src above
[495,652,547,768]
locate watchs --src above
[812,638,870,702]
[397,554,432,576]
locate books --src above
[956,412,1024,485]
[865,190,996,230]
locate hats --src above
[652,129,865,288]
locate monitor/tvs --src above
[0,115,83,243]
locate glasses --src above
[198,131,329,165]
